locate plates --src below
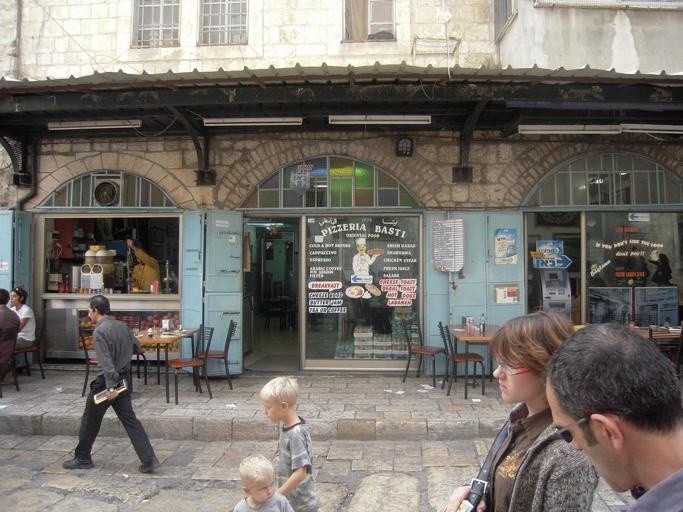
[174,328,189,333]
[133,333,143,338]
[344,286,366,298]
[367,247,386,256]
[643,323,681,334]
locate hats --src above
[355,237,366,246]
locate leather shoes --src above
[62,456,93,470]
[138,458,160,473]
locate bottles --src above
[148,328,153,336]
[479,313,485,332]
[64,275,70,292]
[74,288,113,294]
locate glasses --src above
[551,416,588,443]
[496,356,534,377]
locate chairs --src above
[0,317,20,396]
[74,315,238,403]
[572,313,682,379]
[435,322,487,399]
[401,319,449,388]
[259,291,396,335]
[13,318,47,381]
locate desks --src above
[448,322,505,398]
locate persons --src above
[649,251,677,288]
[229,452,293,512]
[442,307,598,512]
[126,238,162,294]
[61,295,160,475]
[1,287,36,385]
[259,374,319,512]
[0,289,20,364]
[625,247,648,288]
[542,320,682,512]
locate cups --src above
[149,280,160,293]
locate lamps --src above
[328,115,432,126]
[623,124,683,135]
[46,119,143,131]
[516,124,622,135]
[202,116,303,127]
